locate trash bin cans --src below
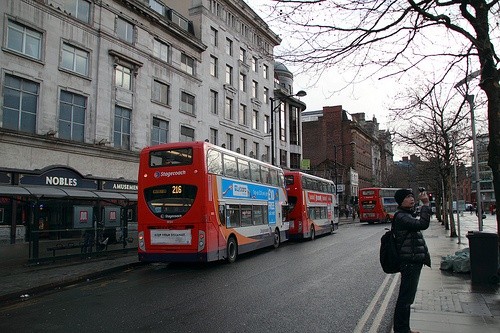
[466,231,498,286]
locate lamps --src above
[97,139,111,146]
[45,130,58,138]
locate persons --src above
[391,188,432,333]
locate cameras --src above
[418,186,425,193]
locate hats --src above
[394,188,414,205]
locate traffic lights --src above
[429,193,433,201]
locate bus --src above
[137,141,290,264]
[358,188,413,224]
[282,171,340,242]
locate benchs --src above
[47,241,95,263]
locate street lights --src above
[269,89,307,166]
[453,69,484,233]
[334,141,357,195]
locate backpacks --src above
[379,210,407,274]
